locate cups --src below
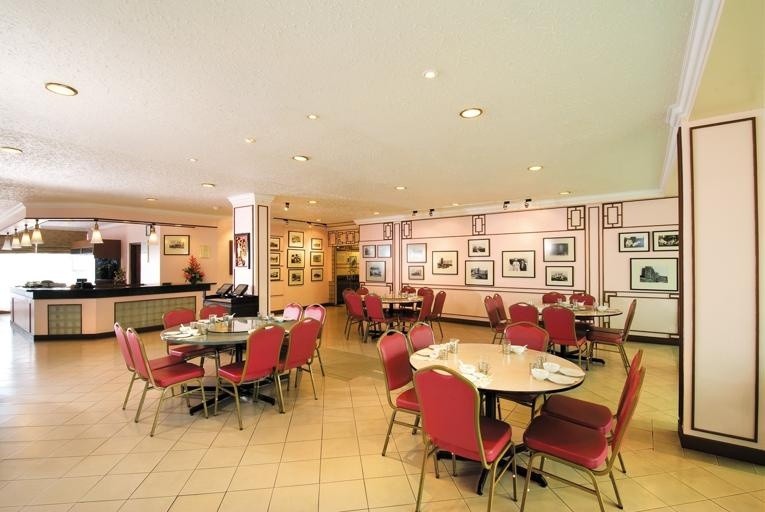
[189,312,275,335]
[529,297,609,312]
[502,338,512,354]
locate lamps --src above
[90,220,104,244]
[1,220,44,252]
[149,224,159,244]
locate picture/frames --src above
[232,230,323,287]
[164,235,190,256]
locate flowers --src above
[182,255,206,283]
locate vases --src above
[190,279,197,284]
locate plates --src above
[415,345,586,385]
[163,331,179,335]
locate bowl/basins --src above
[179,327,190,333]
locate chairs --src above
[112,302,328,436]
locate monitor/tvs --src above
[76,282,94,288]
[216,284,233,295]
[231,284,248,296]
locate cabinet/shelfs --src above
[675,108,764,460]
